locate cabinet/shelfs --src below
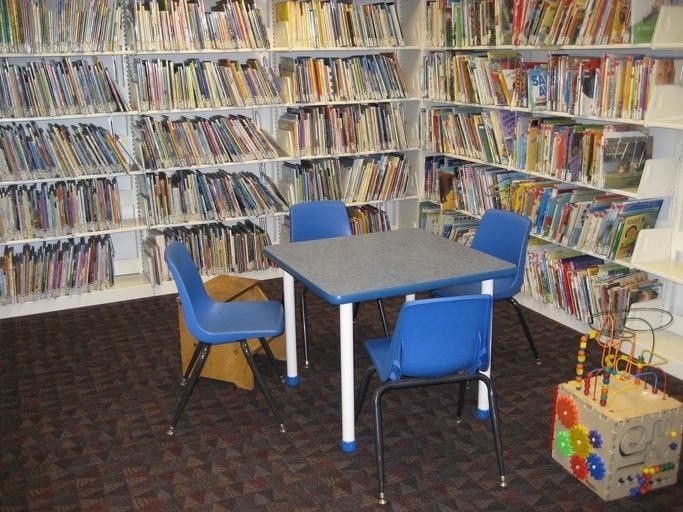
[2,0,421,311]
[422,2,682,382]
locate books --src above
[416,105,653,189]
[425,0,659,46]
[1,1,141,308]
[419,202,663,339]
[420,50,675,114]
[274,1,414,244]
[125,0,293,287]
[423,156,663,261]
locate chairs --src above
[286,198,392,365]
[433,208,540,368]
[163,237,294,439]
[350,294,509,507]
